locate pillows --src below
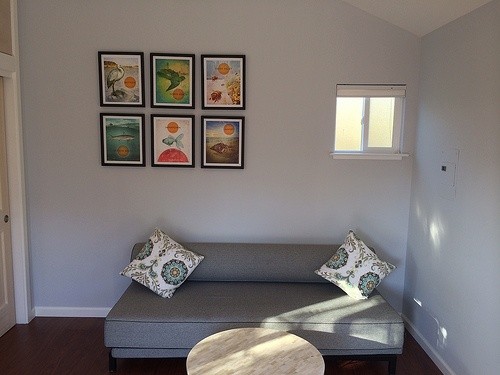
[119,226,204,299]
[314,229,398,300]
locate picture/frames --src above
[150,53,196,109]
[99,112,146,167]
[150,114,195,168]
[98,51,145,108]
[201,54,246,111]
[201,115,245,169]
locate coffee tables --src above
[186,328,326,374]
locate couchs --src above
[103,242,405,375]
